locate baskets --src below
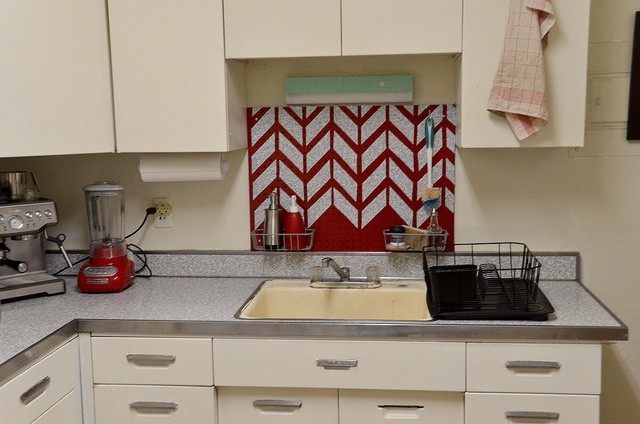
[422,242,541,313]
[383,228,449,251]
[250,229,315,251]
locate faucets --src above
[321,256,351,281]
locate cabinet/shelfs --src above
[456,0,591,149]
[465,332,601,424]
[106,0,248,154]
[0,336,84,423]
[0,0,116,158]
[90,333,219,424]
[223,1,462,60]
[211,339,467,424]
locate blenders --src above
[78,181,136,293]
[0,171,65,302]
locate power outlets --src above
[151,198,173,228]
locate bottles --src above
[427,207,442,249]
[390,227,405,247]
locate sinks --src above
[239,288,427,321]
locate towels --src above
[485,0,558,142]
